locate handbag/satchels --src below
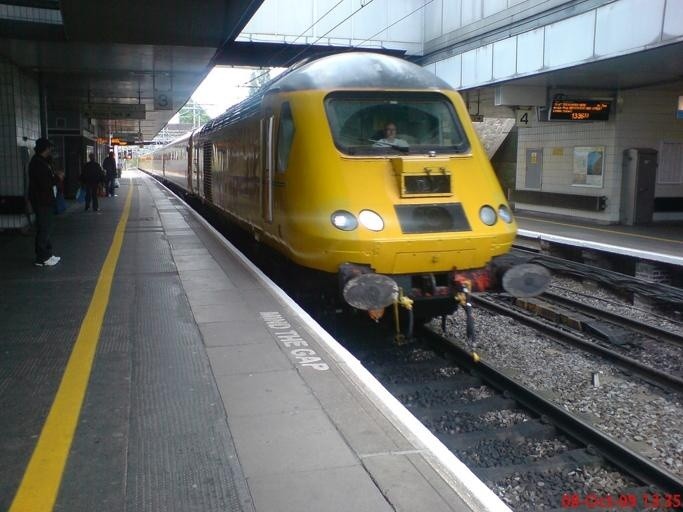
[51,197,65,213]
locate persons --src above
[78,152,104,213]
[102,150,118,198]
[370,120,410,147]
[43,138,57,243]
[26,136,62,267]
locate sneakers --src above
[35,255,60,267]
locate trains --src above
[134,50,521,367]
